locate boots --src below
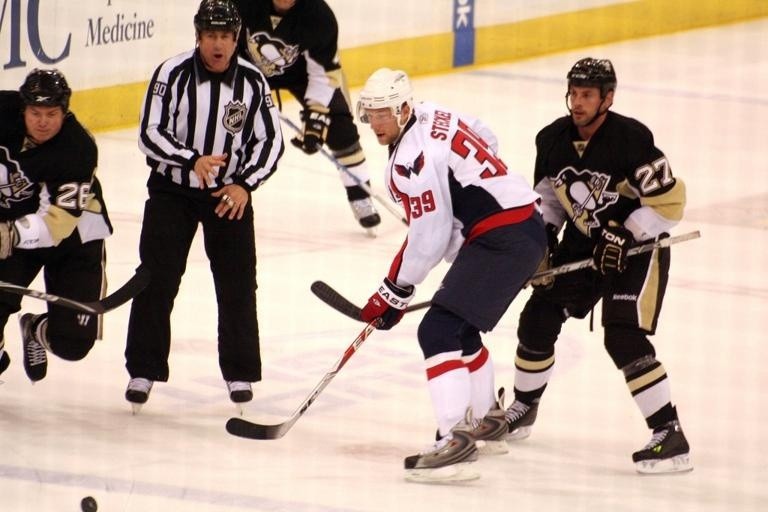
[349,197,381,229]
[404,431,478,470]
[504,400,539,433]
[632,422,690,463]
[226,380,253,402]
[125,377,153,404]
[20,313,47,382]
[435,408,510,442]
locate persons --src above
[232,0,381,229]
[0,66,113,383]
[120,0,286,404]
[502,56,691,465]
[353,64,548,470]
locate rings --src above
[220,194,230,202]
[227,200,235,207]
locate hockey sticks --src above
[311,232,702,324]
[224,316,385,439]
[0,274,135,315]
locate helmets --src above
[18,67,72,114]
[566,57,618,101]
[193,1,242,44]
[354,67,414,131]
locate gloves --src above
[359,277,416,331]
[522,243,556,293]
[591,226,635,278]
[290,106,332,155]
